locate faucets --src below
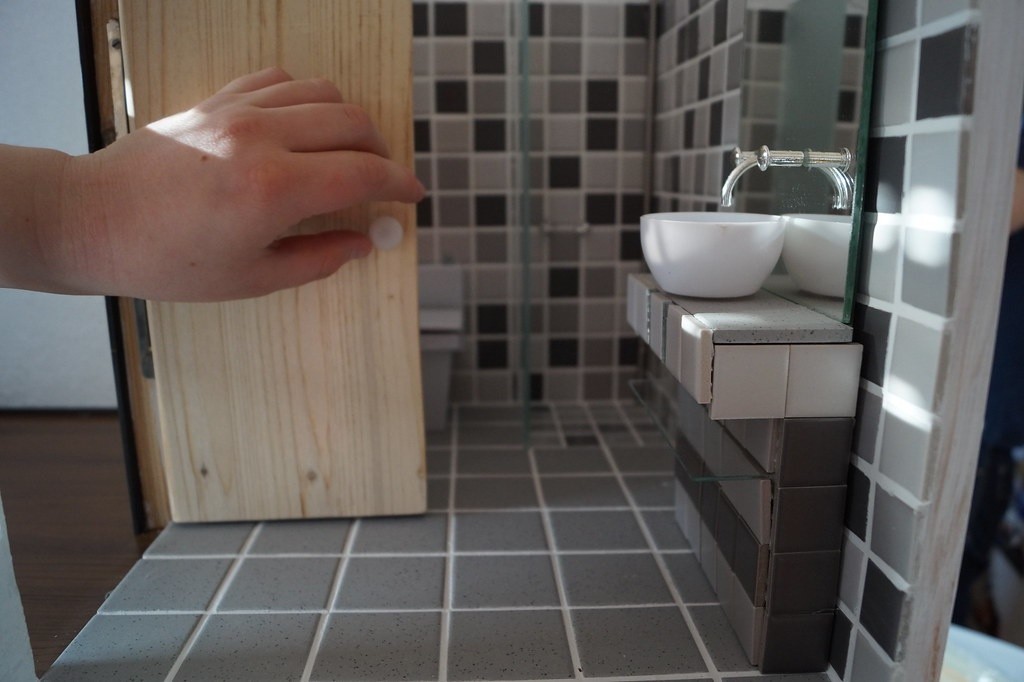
[716,145,803,207]
[802,146,855,210]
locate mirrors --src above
[730,0,878,324]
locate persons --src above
[1,62,426,305]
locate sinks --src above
[786,214,855,299]
[641,214,786,298]
